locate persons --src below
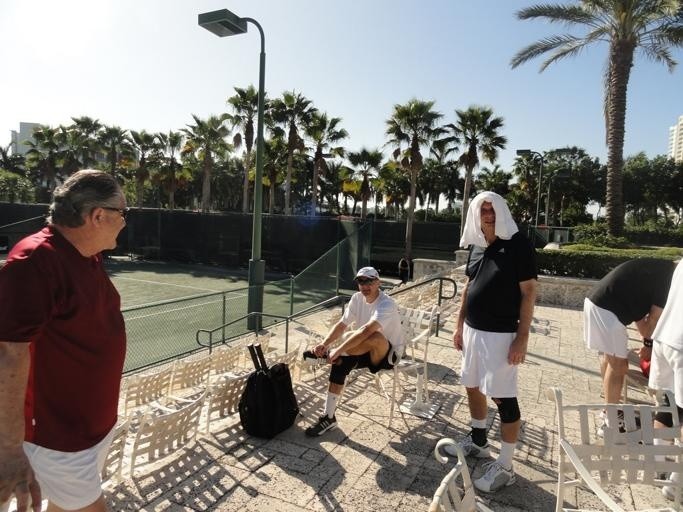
[298,266,408,439]
[581,255,678,436]
[439,192,539,492]
[646,255,683,502]
[0,166,131,510]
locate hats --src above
[353,266,380,281]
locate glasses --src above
[357,279,373,285]
[98,204,130,219]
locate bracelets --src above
[643,337,652,347]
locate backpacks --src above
[238,361,299,440]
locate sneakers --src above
[442,433,490,460]
[655,455,683,504]
[473,460,517,494]
[306,415,338,437]
[597,407,644,445]
[303,349,330,366]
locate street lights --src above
[196,6,268,331]
[516,149,543,225]
